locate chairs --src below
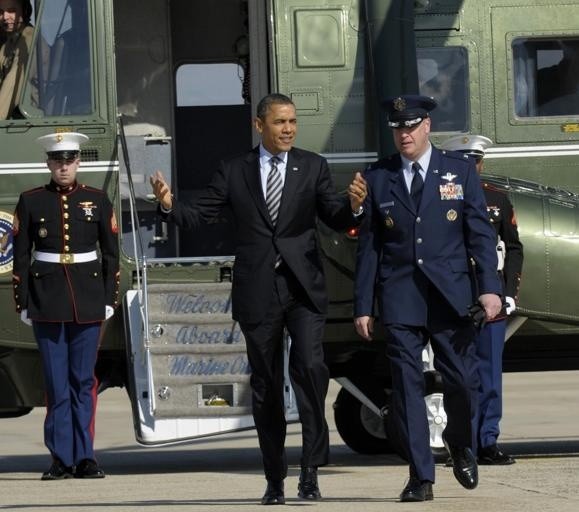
[119,135,174,247]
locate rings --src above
[360,192,364,197]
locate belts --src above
[32,250,98,264]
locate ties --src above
[409,162,425,208]
[265,157,283,269]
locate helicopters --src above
[0,1,578,463]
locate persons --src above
[148,92,367,504]
[352,93,504,502]
[438,130,526,470]
[0,0,55,121]
[11,128,121,482]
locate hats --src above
[441,135,494,162]
[378,93,437,128]
[36,132,89,161]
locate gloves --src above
[505,296,516,316]
[20,309,32,327]
[104,305,115,321]
[466,297,510,330]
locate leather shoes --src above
[442,429,478,489]
[399,473,433,502]
[74,459,106,479]
[297,468,322,502]
[40,457,75,481]
[261,481,285,505]
[476,446,516,465]
